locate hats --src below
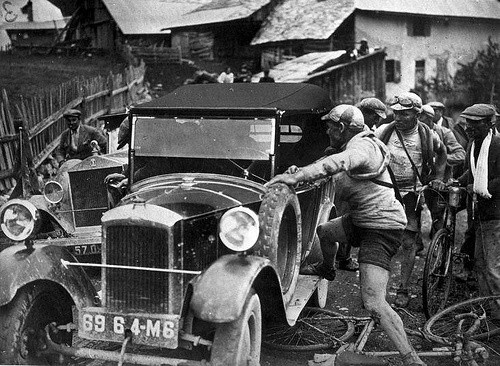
[422,105,436,118]
[426,102,447,112]
[321,104,364,131]
[390,93,423,114]
[361,98,387,120]
[63,110,80,117]
[460,103,496,120]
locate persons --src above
[55,109,108,168]
[259,70,275,83]
[217,67,234,83]
[446,102,500,325]
[334,97,387,271]
[234,64,252,83]
[418,105,466,245]
[427,101,455,132]
[264,104,428,366]
[373,94,447,307]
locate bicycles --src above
[422,180,478,319]
[260,295,499,366]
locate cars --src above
[0,82,339,366]
[30,111,133,241]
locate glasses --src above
[389,96,422,109]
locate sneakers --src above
[394,289,409,308]
[299,260,336,281]
[335,258,359,271]
